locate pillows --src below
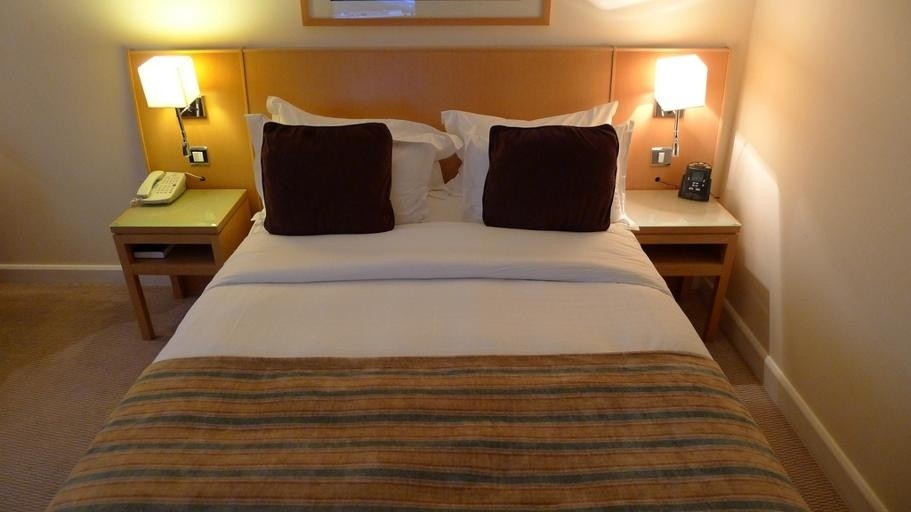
[266,96,463,201]
[441,100,638,233]
[260,122,397,235]
[482,123,619,232]
[244,113,450,225]
[611,117,635,214]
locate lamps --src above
[137,55,201,157]
[653,54,708,158]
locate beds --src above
[45,45,816,512]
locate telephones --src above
[135,170,187,205]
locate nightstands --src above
[109,187,253,339]
[623,187,741,342]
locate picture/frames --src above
[300,0,552,26]
